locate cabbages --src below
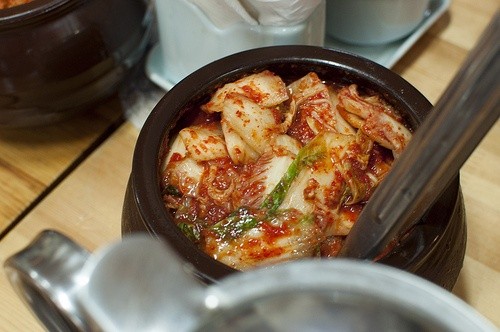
[163,69,413,273]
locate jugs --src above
[5,229,496,332]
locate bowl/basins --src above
[1,1,151,118]
[121,44,466,297]
